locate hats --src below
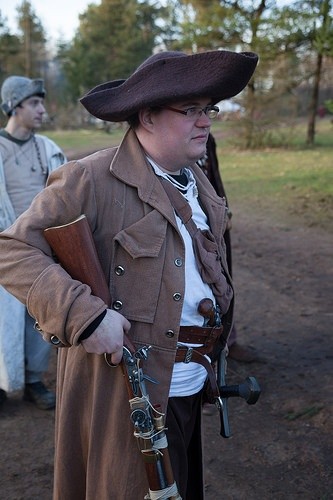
[78,49,259,122]
[0,75,46,116]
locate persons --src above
[0,76,67,410]
[0,51,259,500]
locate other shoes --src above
[24,382,57,412]
[228,342,254,361]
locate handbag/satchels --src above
[156,176,233,313]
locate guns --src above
[197,298,222,326]
[42,214,182,499]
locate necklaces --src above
[14,135,37,172]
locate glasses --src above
[160,104,219,119]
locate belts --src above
[174,343,223,410]
